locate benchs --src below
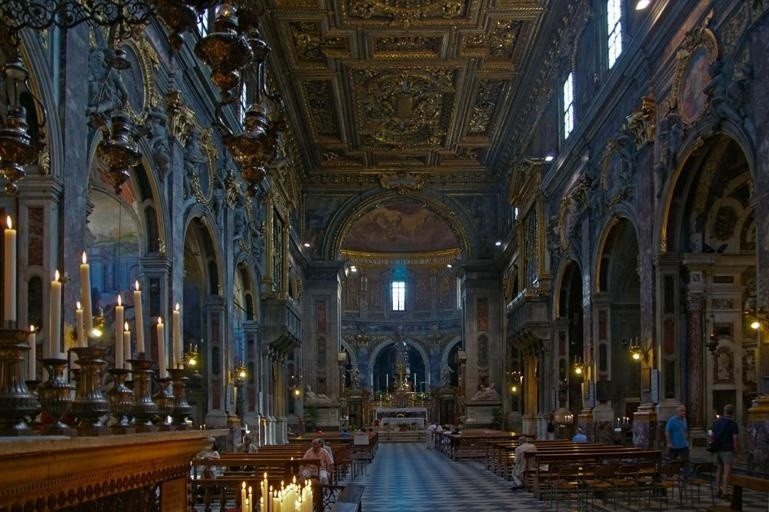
[433,428,534,462]
[482,441,716,508]
[192,431,379,510]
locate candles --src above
[367,371,435,390]
[0,208,192,389]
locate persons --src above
[367,428,376,438]
[318,440,334,479]
[665,405,690,468]
[315,428,325,436]
[426,423,460,449]
[357,428,369,436]
[339,429,350,444]
[300,439,334,506]
[236,436,258,471]
[712,404,739,500]
[510,436,538,490]
[572,427,588,443]
[189,441,220,503]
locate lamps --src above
[570,355,588,380]
[629,336,652,365]
[94,43,143,198]
[213,35,288,200]
[1,34,50,198]
[183,342,201,371]
[742,304,769,339]
[233,366,247,387]
[195,3,256,117]
